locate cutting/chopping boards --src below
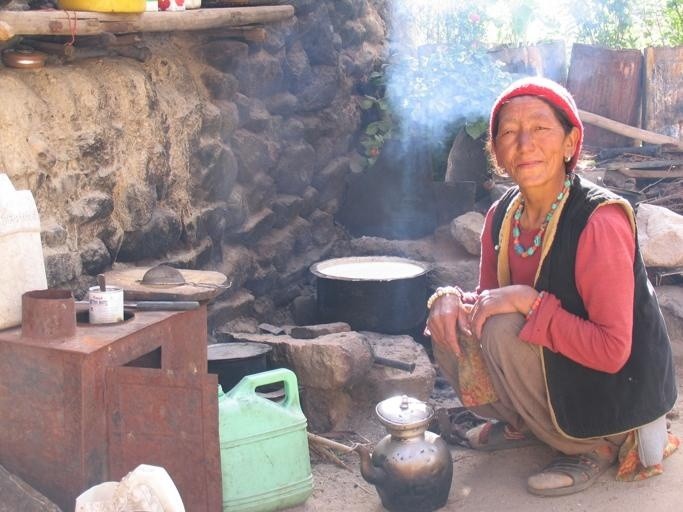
[98,264,232,300]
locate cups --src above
[88,285,125,326]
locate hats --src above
[490,75,584,176]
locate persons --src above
[425,73,679,499]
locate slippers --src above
[466,420,540,449]
[526,448,618,499]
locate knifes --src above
[74,300,201,311]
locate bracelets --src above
[521,286,546,321]
[425,285,462,309]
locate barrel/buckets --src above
[218,368,314,512]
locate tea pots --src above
[346,392,452,512]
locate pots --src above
[308,253,436,335]
[203,340,273,395]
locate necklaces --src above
[511,178,571,260]
